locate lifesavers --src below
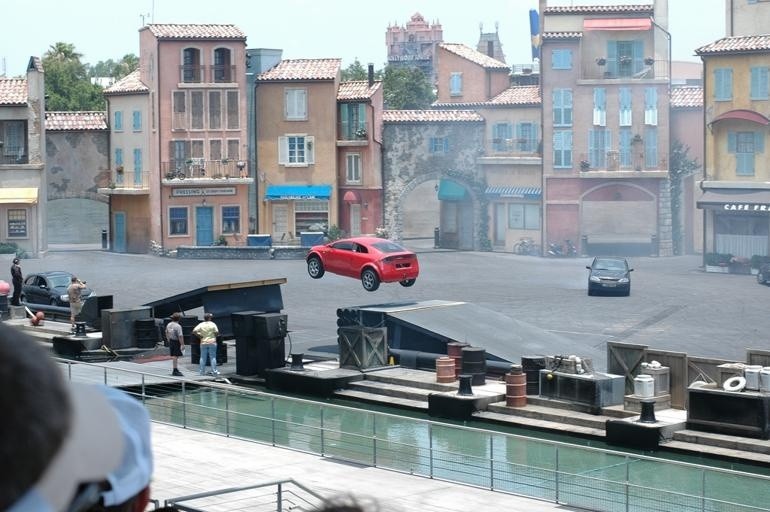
[724,377,746,391]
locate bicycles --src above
[513,237,542,257]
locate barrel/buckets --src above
[164,317,173,347]
[448,343,470,375]
[190,336,227,366]
[0,295,8,321]
[461,347,486,387]
[506,373,527,408]
[521,356,546,395]
[135,318,154,349]
[435,357,455,384]
[179,315,198,345]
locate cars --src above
[586,255,635,297]
[20,271,97,308]
[303,235,420,291]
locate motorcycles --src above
[756,261,770,285]
[549,236,579,258]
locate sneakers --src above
[171,368,183,377]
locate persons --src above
[0,322,73,512]
[8,380,154,512]
[165,313,185,376]
[192,312,221,376]
[67,277,87,326]
[10,258,24,306]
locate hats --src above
[1,321,158,511]
